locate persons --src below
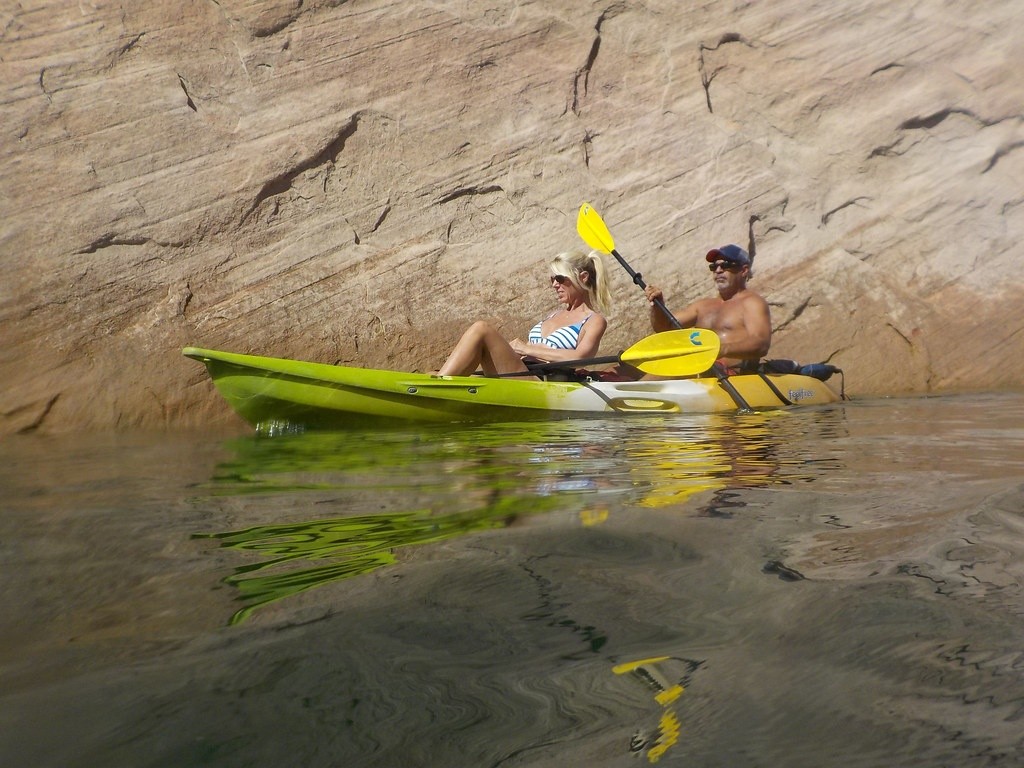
[426,249,612,382]
[638,244,772,381]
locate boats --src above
[182,346,845,439]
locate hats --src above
[706,243,749,264]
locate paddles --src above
[576,202,753,413]
[474,327,721,378]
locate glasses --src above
[709,261,739,271]
[551,275,569,284]
